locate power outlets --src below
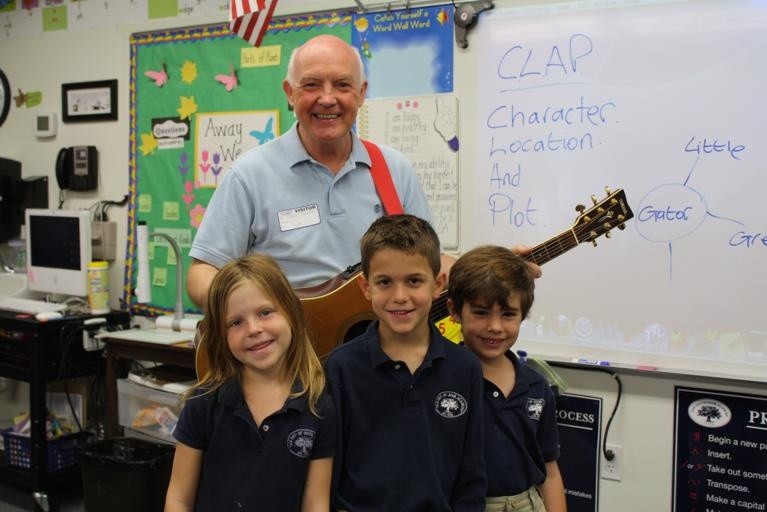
[601,444,625,482]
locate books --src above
[127,361,205,397]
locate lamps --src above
[135,221,198,331]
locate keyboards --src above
[0,296,68,315]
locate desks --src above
[0,308,130,512]
[103,337,197,434]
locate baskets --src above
[0,427,84,472]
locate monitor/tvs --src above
[23,207,92,303]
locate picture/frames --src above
[61,78,118,124]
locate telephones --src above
[55,145,99,191]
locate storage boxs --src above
[116,377,182,444]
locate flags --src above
[226,0,277,47]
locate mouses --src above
[35,312,63,322]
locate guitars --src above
[193,188,633,381]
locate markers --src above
[571,358,609,365]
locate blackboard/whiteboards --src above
[453,1,766,387]
[73,437,176,512]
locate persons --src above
[184,33,543,309]
[448,245,568,512]
[164,256,336,511]
[322,214,488,511]
